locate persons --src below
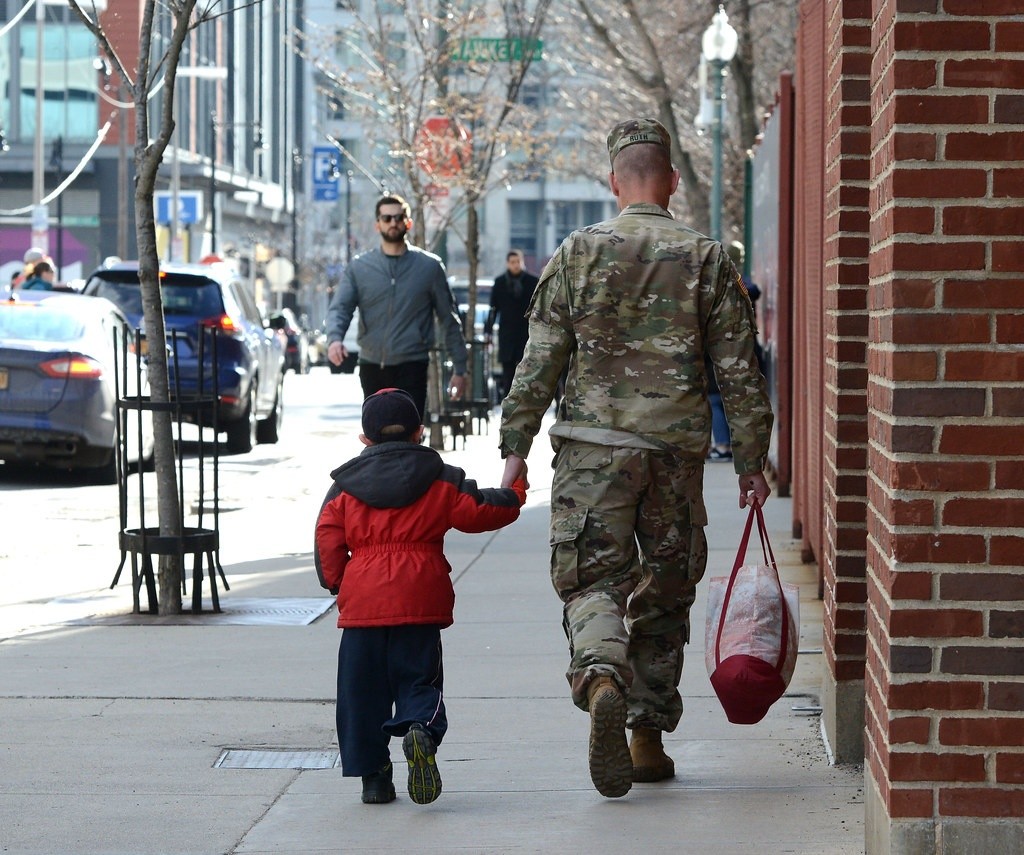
[495,116,775,798]
[707,279,762,462]
[483,250,538,394]
[10,248,58,290]
[313,389,528,805]
[326,195,467,423]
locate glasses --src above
[378,214,407,223]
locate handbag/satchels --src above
[705,496,799,725]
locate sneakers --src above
[362,762,396,804]
[402,729,442,804]
[704,446,733,462]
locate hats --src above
[607,118,674,175]
[24,247,46,264]
[362,388,421,443]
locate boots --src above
[588,676,633,797]
[627,723,674,782]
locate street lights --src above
[700,2,738,249]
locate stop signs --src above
[411,118,475,178]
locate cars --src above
[261,308,313,375]
[450,278,500,334]
[0,289,155,485]
[325,306,362,375]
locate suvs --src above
[81,256,289,456]
